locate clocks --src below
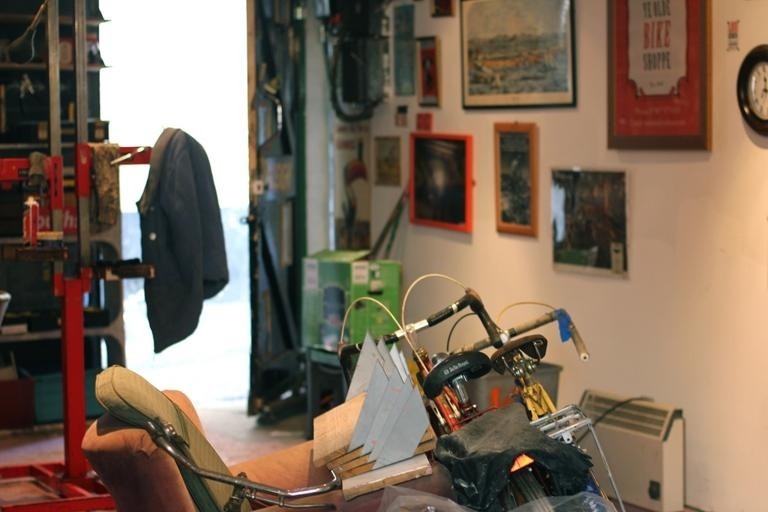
[734,42,766,137]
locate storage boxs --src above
[301,247,402,355]
[0,358,104,430]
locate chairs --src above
[78,357,455,509]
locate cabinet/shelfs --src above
[0,0,122,439]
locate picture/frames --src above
[460,0,578,110]
[494,123,539,237]
[606,0,713,151]
[429,2,454,17]
[409,132,474,234]
[413,36,442,107]
[550,167,630,276]
